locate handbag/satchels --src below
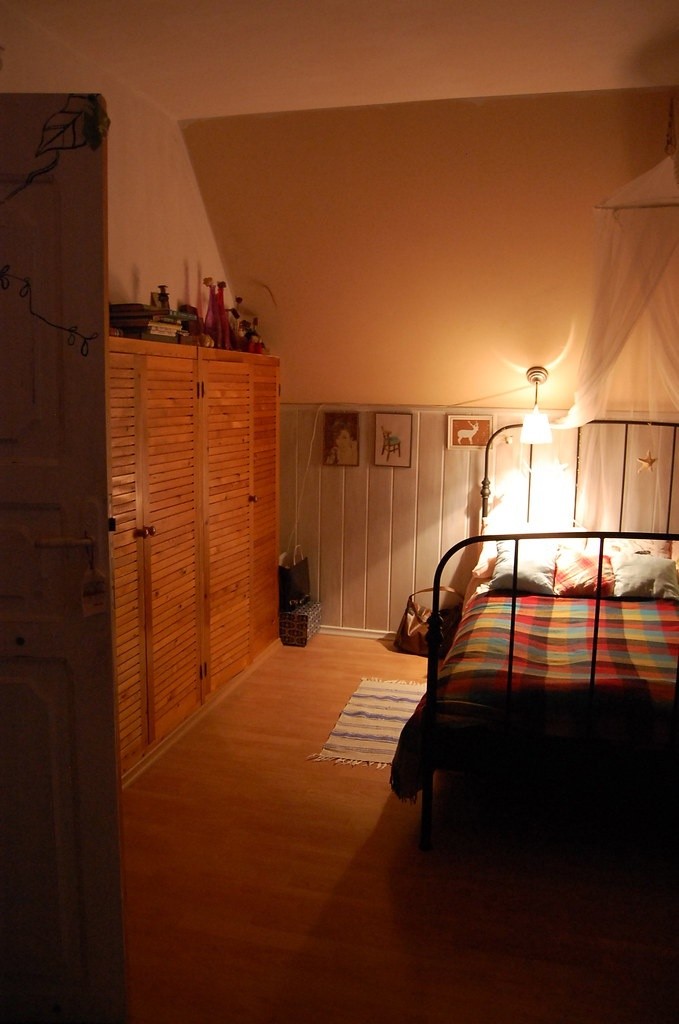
[278,544,310,611]
[393,586,464,656]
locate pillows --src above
[610,556,679,601]
[471,515,589,577]
[488,540,561,595]
[553,550,615,597]
[587,531,672,558]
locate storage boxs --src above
[279,600,324,647]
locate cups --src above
[249,336,262,352]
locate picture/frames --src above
[446,414,494,450]
[322,410,360,467]
[374,412,413,468]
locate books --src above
[108,303,197,344]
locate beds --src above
[390,420,678,853]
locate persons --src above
[325,419,358,464]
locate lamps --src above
[520,366,554,445]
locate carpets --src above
[311,676,426,767]
